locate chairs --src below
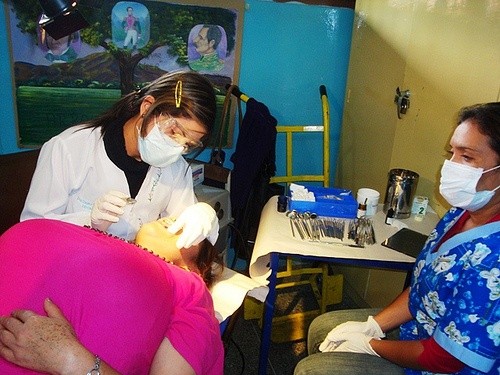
[0,150,40,235]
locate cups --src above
[356,188,380,219]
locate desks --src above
[249,195,442,375]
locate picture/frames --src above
[4,0,244,149]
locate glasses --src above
[161,112,204,159]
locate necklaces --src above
[83,225,175,264]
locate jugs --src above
[383,168,420,218]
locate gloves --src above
[90,190,131,233]
[319,315,386,354]
[330,334,381,359]
[168,201,218,249]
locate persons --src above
[294,101,500,375]
[0,215,227,375]
[20,68,220,249]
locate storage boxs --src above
[288,183,359,218]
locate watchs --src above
[87,355,101,375]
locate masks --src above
[439,159,500,212]
[136,114,184,168]
